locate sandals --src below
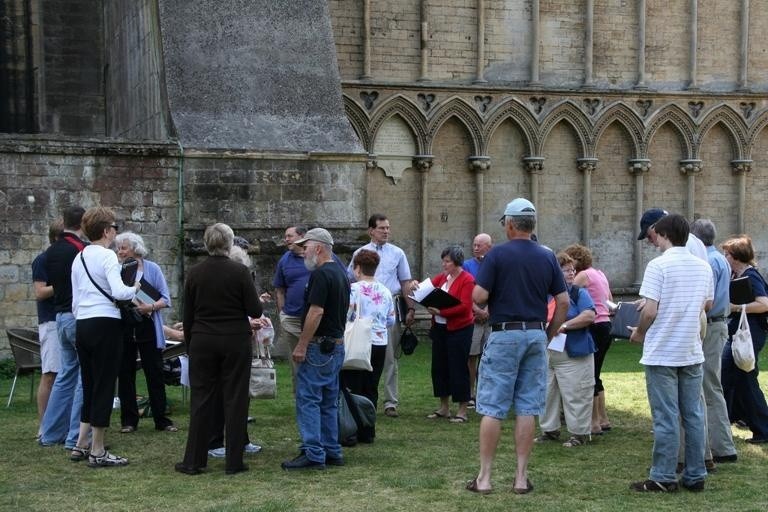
[71,426,137,467]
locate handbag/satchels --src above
[343,319,374,372]
[400,327,417,355]
[731,329,755,373]
[116,300,142,328]
[249,359,277,399]
[135,394,171,417]
[338,388,376,446]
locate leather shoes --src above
[166,425,178,432]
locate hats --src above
[638,209,669,240]
[499,198,536,222]
[294,228,334,247]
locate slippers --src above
[467,476,490,494]
[514,478,533,493]
[426,412,467,423]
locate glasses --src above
[112,224,118,231]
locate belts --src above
[492,322,541,331]
[707,316,723,322]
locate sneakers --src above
[533,427,610,447]
[385,407,398,417]
[175,442,262,475]
[281,454,344,470]
[630,454,737,492]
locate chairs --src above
[3,324,43,408]
[154,338,191,407]
